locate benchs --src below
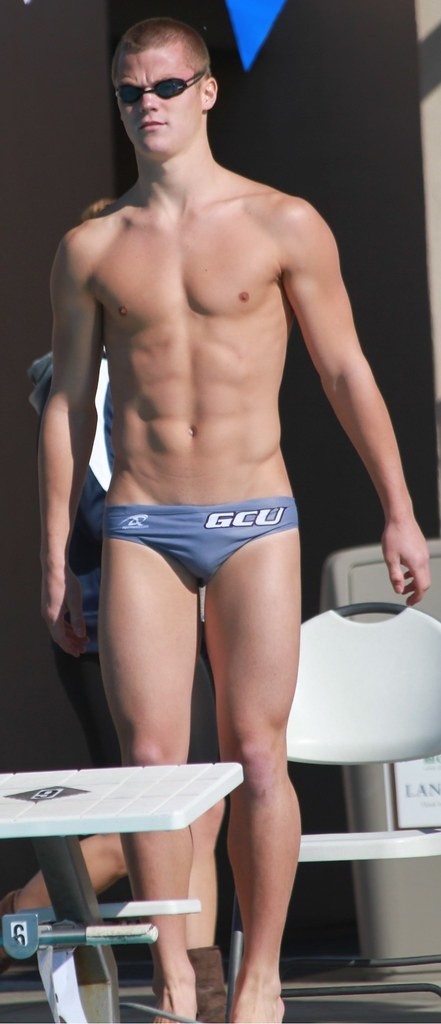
[0,897,203,945]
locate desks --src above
[0,761,245,1024]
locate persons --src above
[0,16,431,1024]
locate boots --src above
[187,944,227,1023]
[0,891,18,975]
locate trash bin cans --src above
[319,537,441,971]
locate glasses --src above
[115,68,208,104]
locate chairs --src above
[224,601,441,1024]
